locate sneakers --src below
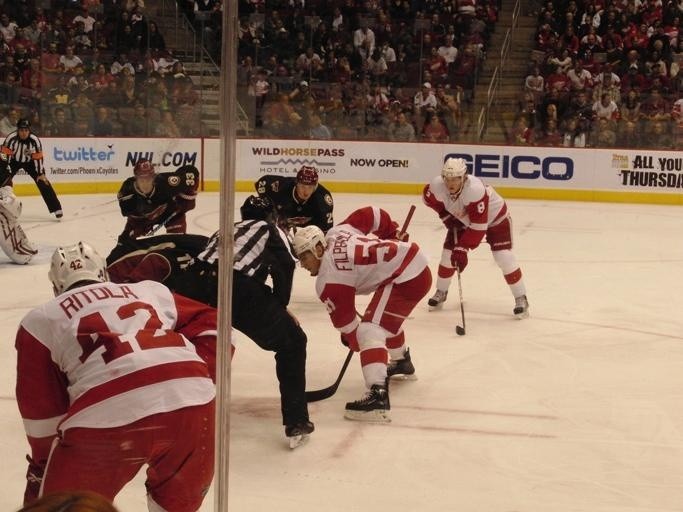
[345,377,392,412]
[55,210,64,218]
[386,347,416,376]
[285,421,315,438]
[429,288,449,307]
[513,295,530,316]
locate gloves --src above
[421,184,445,214]
[451,246,470,274]
[396,231,410,243]
[339,330,362,353]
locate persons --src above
[14,239,234,512]
[102,153,211,282]
[0,119,63,220]
[515,1,683,152]
[0,185,38,264]
[179,0,501,144]
[291,206,431,413]
[423,156,528,316]
[254,165,334,237]
[180,195,315,437]
[0,0,209,138]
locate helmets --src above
[177,165,199,200]
[17,118,31,129]
[239,196,275,222]
[297,166,319,186]
[48,238,109,296]
[134,158,157,179]
[292,225,328,257]
[440,157,468,179]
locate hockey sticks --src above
[305,204,416,402]
[453,224,465,336]
